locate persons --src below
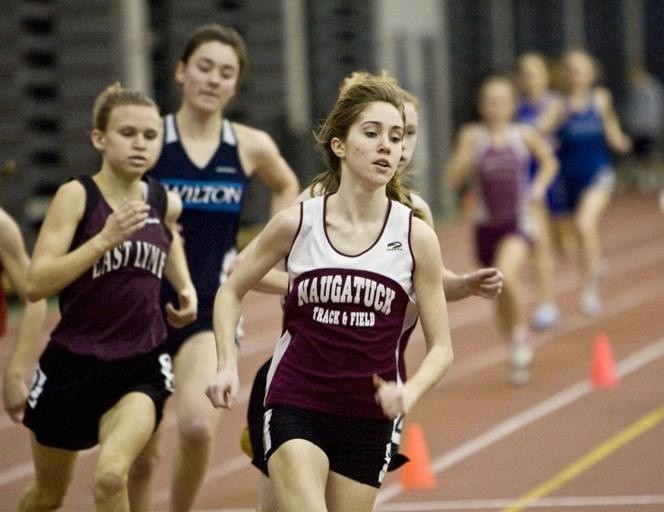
[446,47,664,384]
[0,24,504,512]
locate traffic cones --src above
[588,331,620,392]
[398,419,443,493]
